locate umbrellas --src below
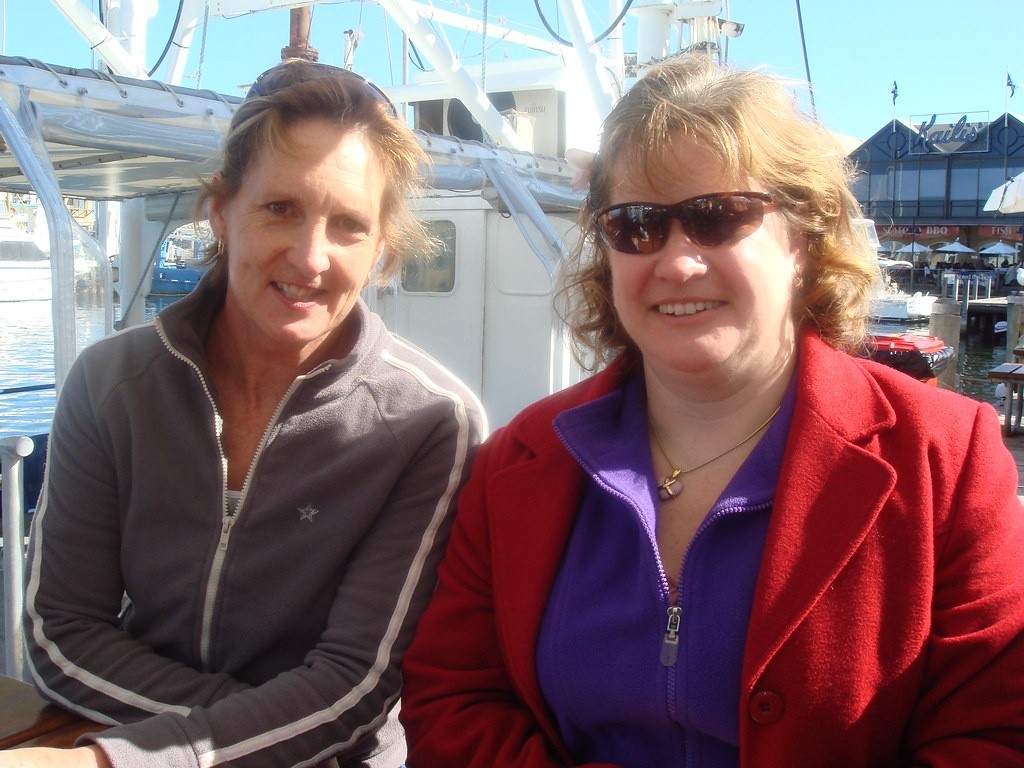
[877,241,1020,269]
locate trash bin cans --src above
[843,335,944,388]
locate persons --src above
[402,51,1024,768]
[0,56,489,768]
[915,259,1024,283]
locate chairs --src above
[890,259,1018,291]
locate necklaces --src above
[649,407,780,503]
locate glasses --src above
[593,192,772,254]
